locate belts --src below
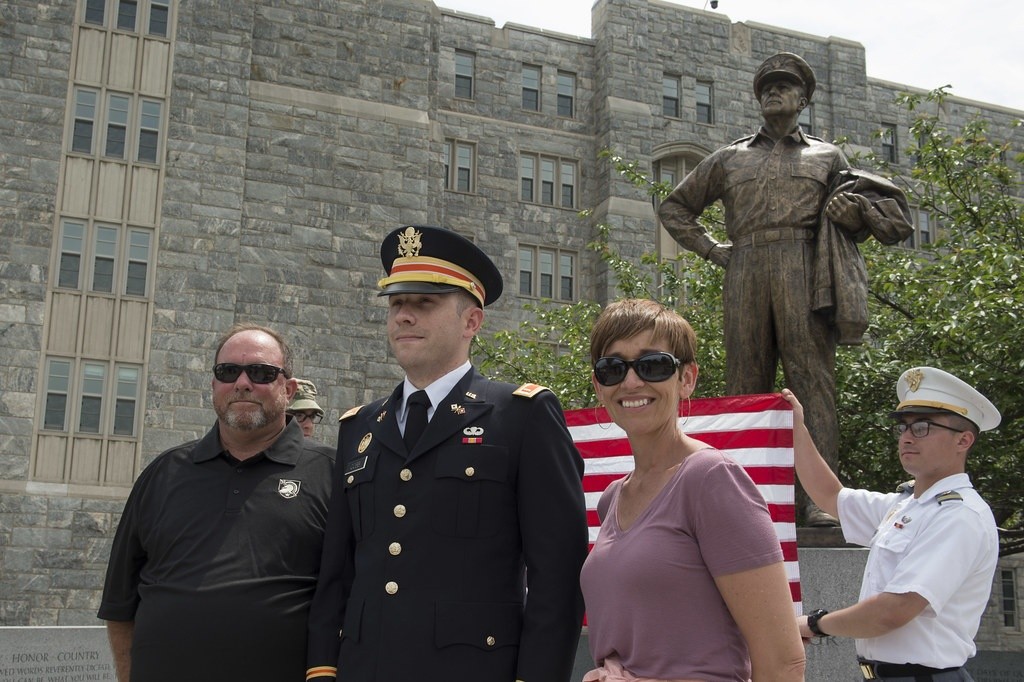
[857,660,958,680]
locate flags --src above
[563,393,802,617]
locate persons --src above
[659,52,872,526]
[285,378,324,438]
[307,226,589,682]
[580,301,805,682]
[97,327,337,682]
[782,367,1001,682]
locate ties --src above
[399,390,431,454]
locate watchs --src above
[807,609,830,637]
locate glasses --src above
[211,362,288,384]
[291,411,323,425]
[593,352,681,387]
[889,419,968,439]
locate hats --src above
[896,367,1002,433]
[377,224,504,309]
[285,378,325,415]
